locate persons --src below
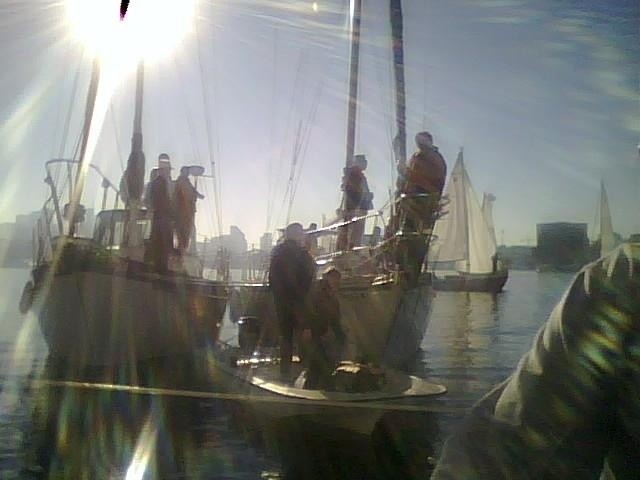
[344,152,374,251]
[491,251,500,272]
[390,132,449,286]
[431,238,639,478]
[303,223,318,252]
[119,135,204,269]
[269,222,317,381]
[291,267,346,390]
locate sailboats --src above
[428,146,509,295]
